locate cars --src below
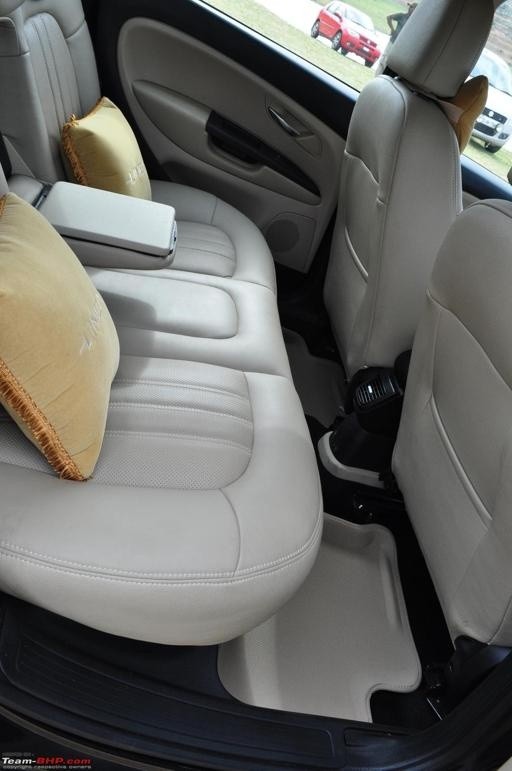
[311,0,512,153]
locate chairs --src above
[1,1,512,652]
